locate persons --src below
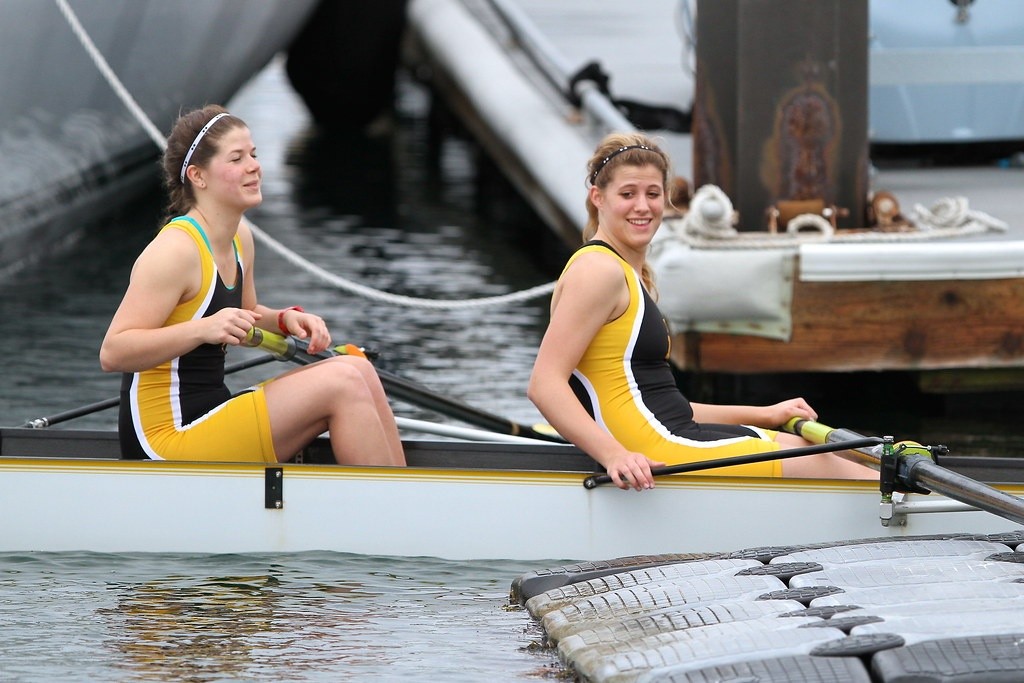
[527,135,880,492]
[100,105,406,467]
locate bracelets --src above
[278,306,304,336]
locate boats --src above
[0,425,1024,557]
